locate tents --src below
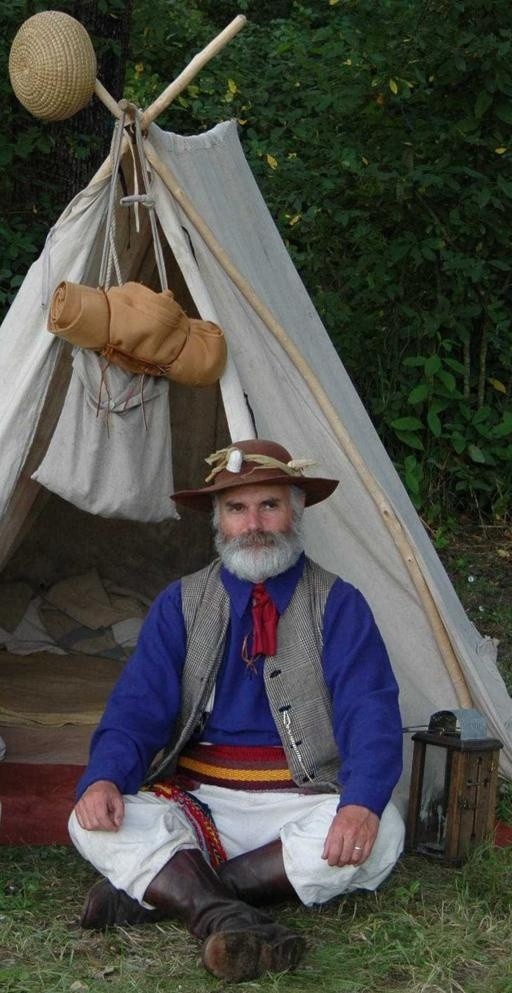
[0,112,512,820]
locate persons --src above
[68,441,405,985]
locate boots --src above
[79,836,305,984]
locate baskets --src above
[8,10,97,121]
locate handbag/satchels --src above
[29,346,183,527]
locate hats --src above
[169,438,340,512]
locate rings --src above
[354,847,363,850]
